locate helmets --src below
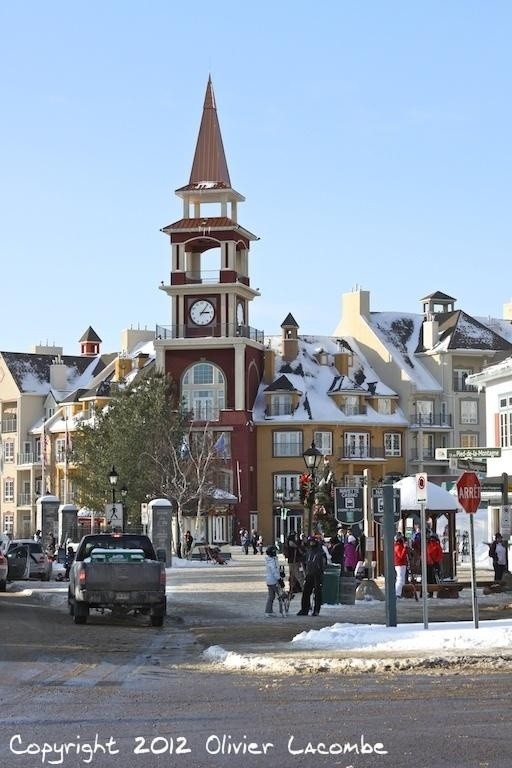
[267,547,276,555]
[307,536,320,543]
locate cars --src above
[0,537,80,592]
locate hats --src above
[290,530,298,535]
[331,536,339,544]
[394,532,403,542]
[495,532,501,538]
[347,535,356,543]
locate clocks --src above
[189,299,215,326]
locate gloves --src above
[280,571,285,578]
[278,578,285,588]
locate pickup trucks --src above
[67,532,167,627]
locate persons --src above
[489,532,508,581]
[34,528,56,554]
[239,526,444,617]
[185,531,193,553]
[66,546,75,579]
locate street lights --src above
[120,484,129,522]
[300,439,325,535]
[108,467,121,505]
[274,488,296,539]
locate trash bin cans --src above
[321,563,341,605]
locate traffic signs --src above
[455,471,482,514]
[447,457,486,473]
[479,482,501,492]
[434,444,503,460]
[413,471,429,505]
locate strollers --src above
[202,544,228,566]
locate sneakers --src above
[297,610,308,615]
[265,613,277,617]
[312,611,319,616]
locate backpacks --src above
[284,541,290,557]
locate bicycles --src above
[276,564,294,616]
